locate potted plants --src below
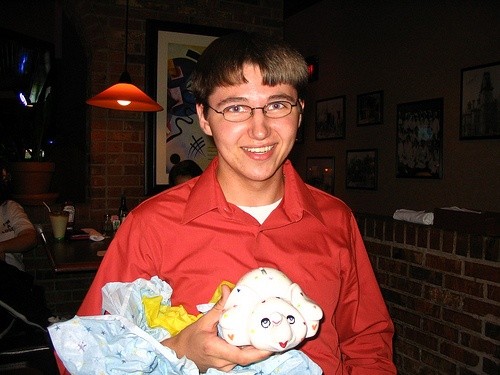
[10,48,55,194]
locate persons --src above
[396,110,441,176]
[169,159,203,188]
[55,29,397,375]
[0,165,37,340]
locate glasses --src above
[208,98,298,123]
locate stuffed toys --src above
[218,266,323,352]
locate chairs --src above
[0,298,53,364]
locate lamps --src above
[86,0,164,112]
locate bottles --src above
[119,194,128,220]
[102,213,113,236]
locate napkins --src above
[81,228,104,241]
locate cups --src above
[63,205,75,230]
[48,211,69,240]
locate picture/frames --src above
[344,148,379,191]
[459,61,500,140]
[394,96,444,179]
[356,89,384,127]
[314,95,346,141]
[306,155,336,195]
[143,17,248,197]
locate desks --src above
[40,231,110,273]
